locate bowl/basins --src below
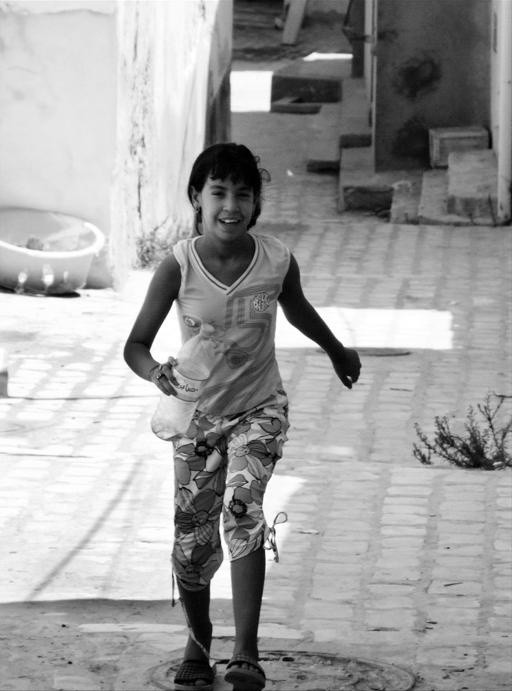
[0,207,106,296]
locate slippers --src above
[173,657,219,690]
[224,655,267,690]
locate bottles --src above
[149,322,216,443]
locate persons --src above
[121,143,363,689]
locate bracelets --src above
[148,364,160,381]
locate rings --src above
[156,373,168,382]
[158,364,171,372]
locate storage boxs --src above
[428,125,490,169]
